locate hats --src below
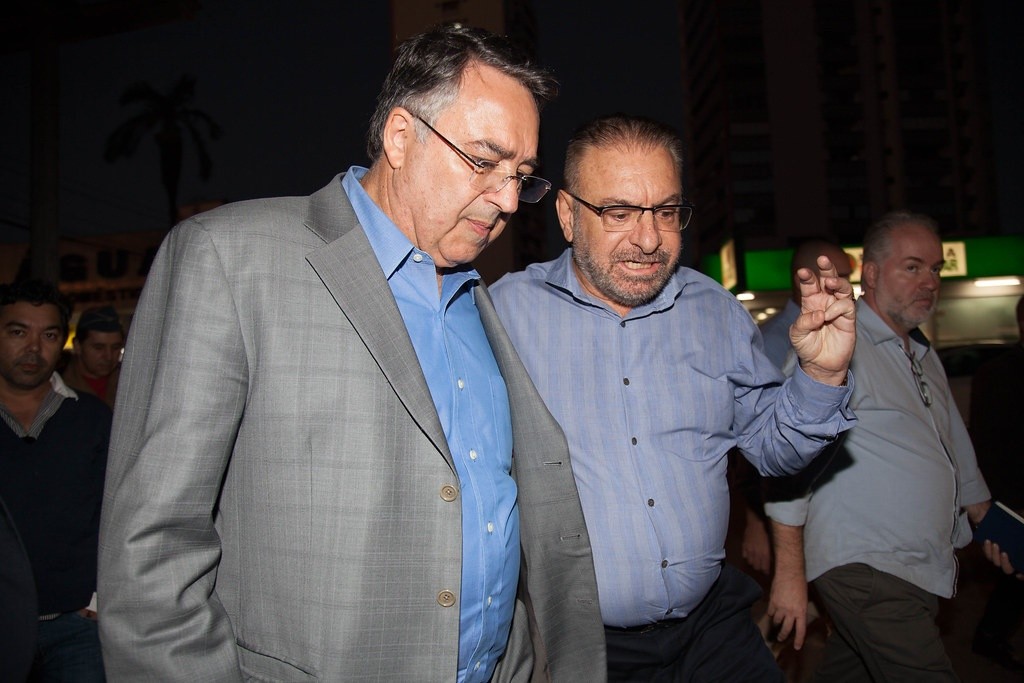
[77,304,120,325]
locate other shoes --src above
[972,637,1021,670]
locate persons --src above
[732,209,1023,683]
[95,22,608,683]
[482,112,858,683]
[0,274,125,683]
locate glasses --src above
[405,107,552,204]
[908,349,933,408]
[563,190,693,231]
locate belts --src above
[611,619,670,636]
